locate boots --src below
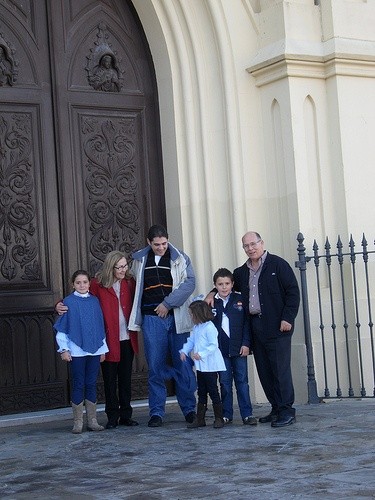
[187,404,207,428]
[213,403,225,427]
[85,398,104,431]
[71,400,84,433]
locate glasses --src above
[114,264,128,270]
[243,240,260,249]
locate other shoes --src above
[223,417,229,424]
[119,418,138,426]
[106,418,117,428]
[243,416,257,424]
[148,416,162,427]
[186,412,197,424]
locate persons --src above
[55,250,138,428]
[180,300,227,429]
[53,269,109,433]
[130,224,197,426]
[204,231,300,427]
[210,268,258,425]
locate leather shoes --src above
[259,412,273,423]
[271,416,296,426]
